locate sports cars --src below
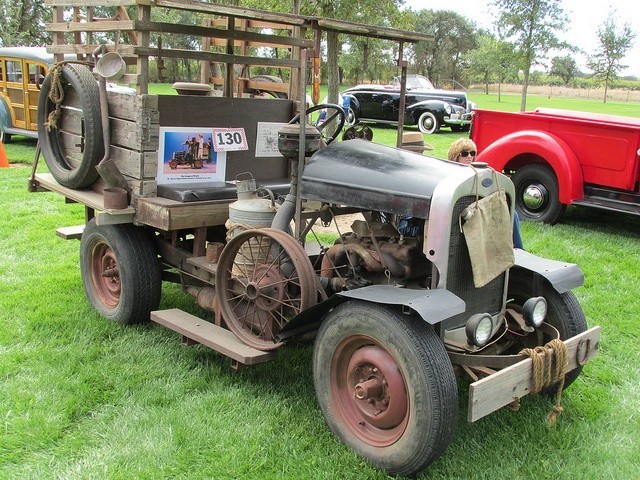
[340,75,476,135]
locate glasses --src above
[460,151,476,156]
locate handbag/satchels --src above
[459,187,515,288]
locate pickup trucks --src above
[469,106,639,225]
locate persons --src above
[447,136,480,164]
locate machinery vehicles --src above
[27,0,600,476]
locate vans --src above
[0,47,102,144]
